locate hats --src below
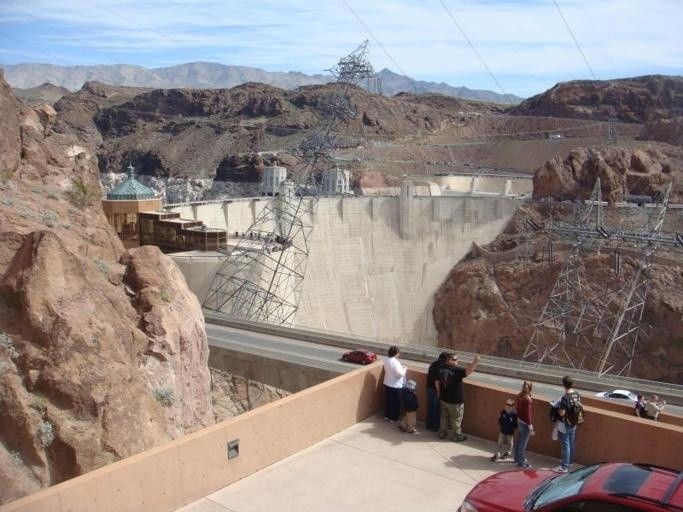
[405,380,416,389]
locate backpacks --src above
[562,391,584,427]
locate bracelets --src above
[529,429,534,432]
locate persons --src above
[433,352,481,442]
[487,398,517,462]
[634,394,647,418]
[381,344,408,423]
[645,394,666,421]
[396,379,418,433]
[425,351,448,431]
[514,380,536,468]
[550,375,581,474]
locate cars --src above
[341,347,378,365]
[592,387,641,403]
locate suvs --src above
[451,457,682,512]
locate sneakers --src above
[438,430,467,441]
[385,416,418,434]
[551,460,573,474]
[491,451,533,469]
